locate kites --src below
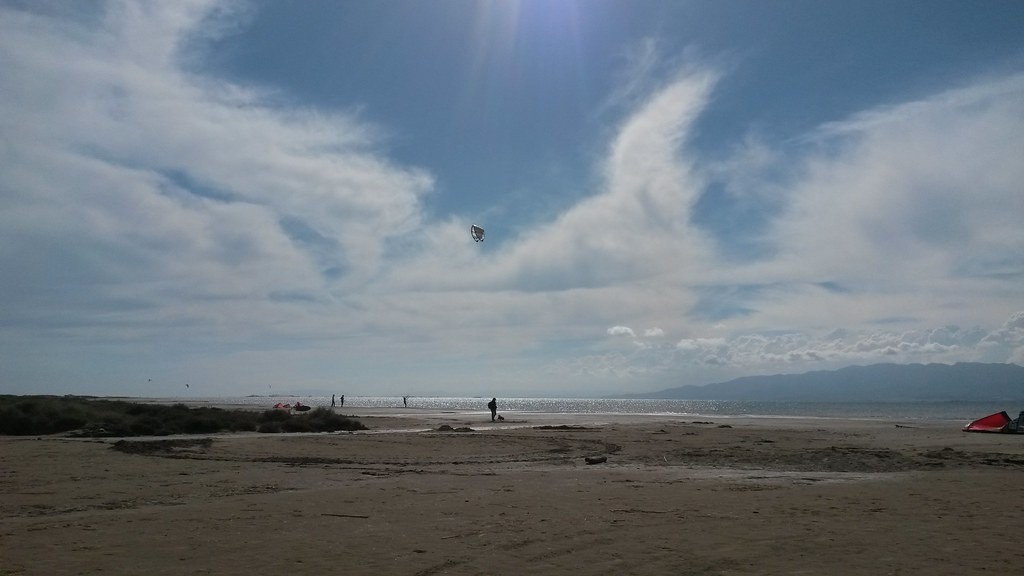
[470,224,485,242]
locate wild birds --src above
[184,384,190,389]
[148,378,152,382]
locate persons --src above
[341,395,344,407]
[331,394,335,407]
[491,398,497,421]
[404,397,406,407]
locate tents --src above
[966,411,1012,431]
